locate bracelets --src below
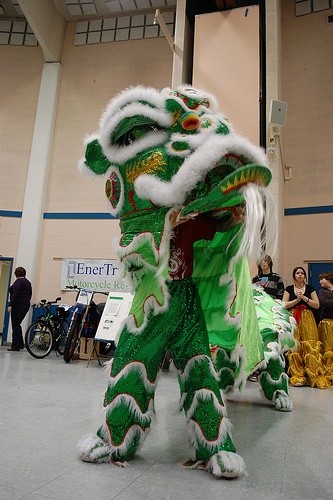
[306,299,309,303]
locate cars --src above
[24,296,70,359]
[61,285,113,363]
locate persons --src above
[316,271,333,386]
[282,267,328,389]
[214,332,295,412]
[250,253,285,382]
[7,267,32,351]
[80,213,246,478]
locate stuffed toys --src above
[80,84,298,391]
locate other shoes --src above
[295,382,305,386]
[8,349,20,351]
[250,377,258,381]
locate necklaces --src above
[295,283,304,289]
